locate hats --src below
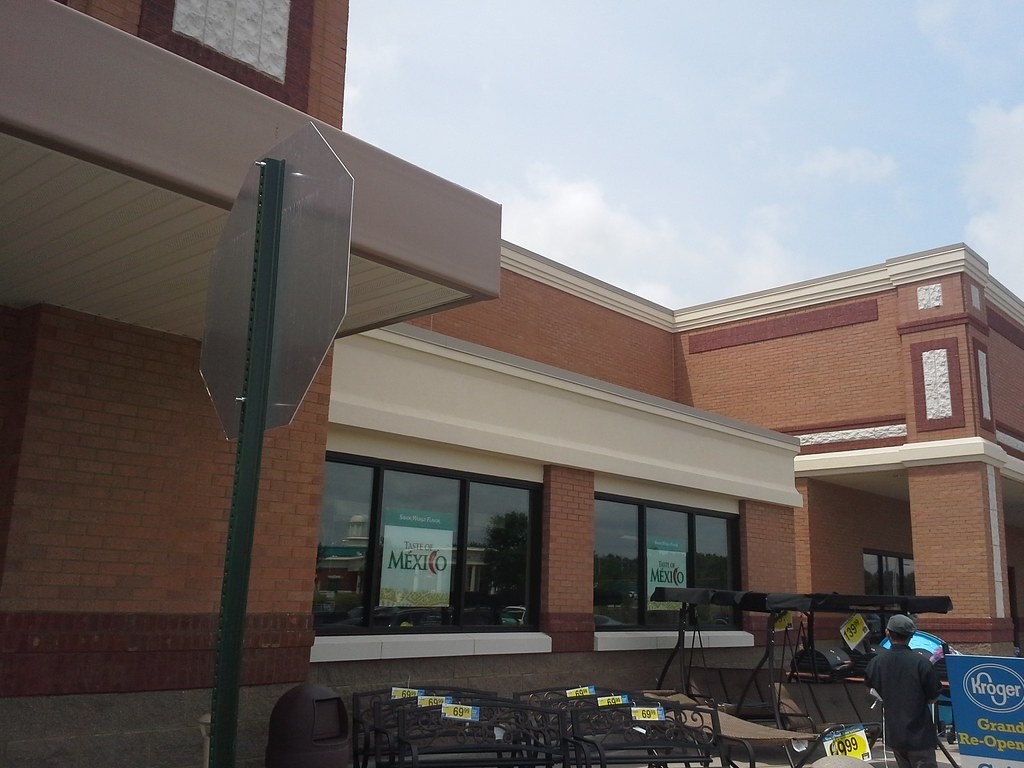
[887,614,917,638]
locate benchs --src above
[772,681,884,742]
[684,667,788,718]
[350,684,755,768]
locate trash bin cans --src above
[265,683,352,768]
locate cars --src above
[503,606,527,624]
[326,606,498,627]
[594,615,623,626]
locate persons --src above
[863,614,943,768]
